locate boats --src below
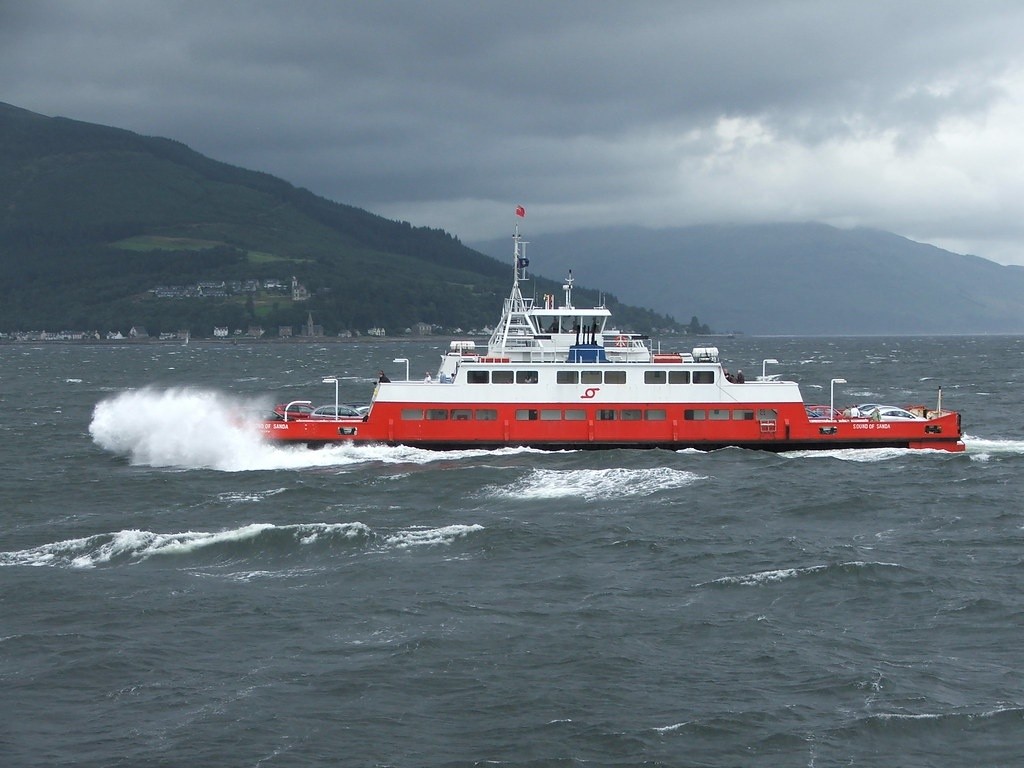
[221,206,966,454]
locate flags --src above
[516,205,524,217]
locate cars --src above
[274,402,315,419]
[354,406,369,414]
[804,405,843,419]
[850,403,927,421]
[228,407,285,421]
[310,404,362,421]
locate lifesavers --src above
[614,336,627,347]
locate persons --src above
[548,318,567,333]
[450,373,456,384]
[378,370,390,383]
[440,371,447,383]
[424,372,432,383]
[843,404,881,422]
[724,369,744,384]
